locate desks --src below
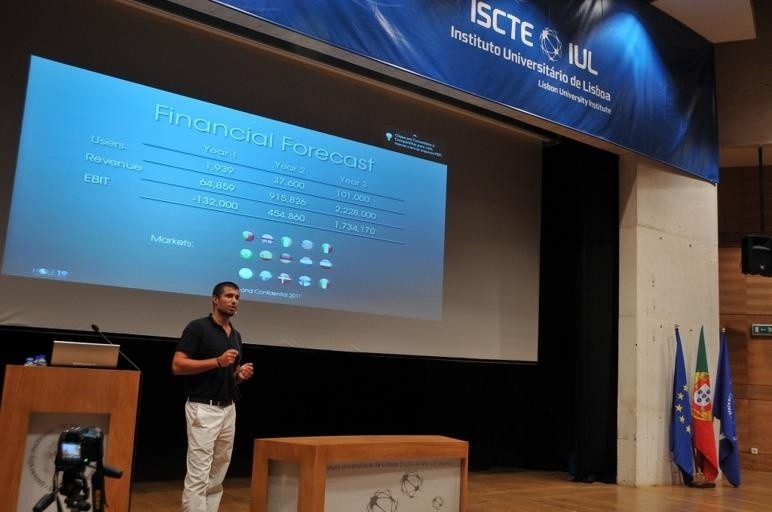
[250,434,470,510]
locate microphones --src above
[91,324,139,370]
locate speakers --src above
[741,234,772,277]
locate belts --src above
[187,394,237,407]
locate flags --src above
[689,324,719,486]
[665,322,696,487]
[710,327,742,488]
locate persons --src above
[168,281,257,511]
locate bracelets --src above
[216,356,224,370]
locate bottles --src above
[25,353,48,366]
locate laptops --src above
[51,340,121,368]
